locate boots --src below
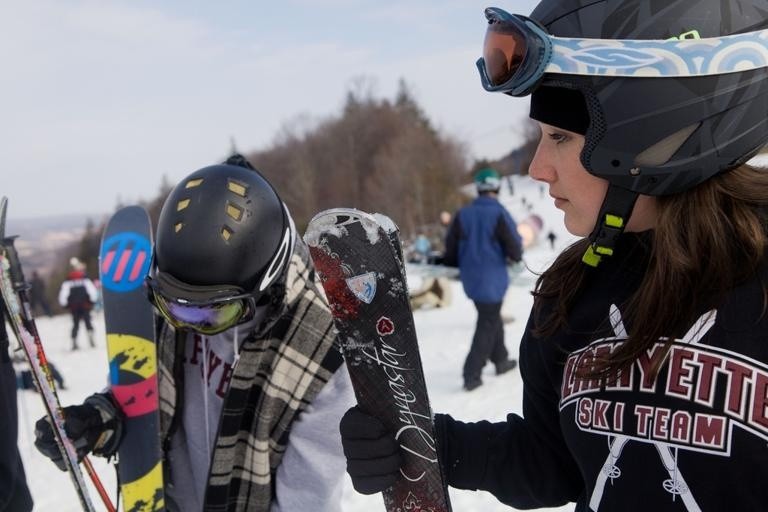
[70,328,97,352]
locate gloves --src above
[338,400,453,496]
[35,391,124,472]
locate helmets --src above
[474,168,501,194]
[522,1,768,199]
[153,164,291,290]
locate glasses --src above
[150,272,250,334]
[476,6,551,100]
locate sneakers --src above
[462,359,517,392]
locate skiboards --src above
[304,207,452,512]
[0,193,167,512]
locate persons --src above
[342,0,768,510]
[34,161,383,512]
